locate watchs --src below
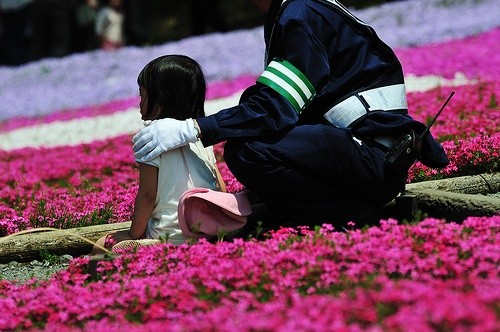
[192,119,199,137]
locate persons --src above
[93,54,226,253]
[77,0,124,50]
[132,0,449,232]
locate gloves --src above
[131,117,199,161]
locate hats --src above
[178,186,251,237]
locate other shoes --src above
[113,238,160,251]
